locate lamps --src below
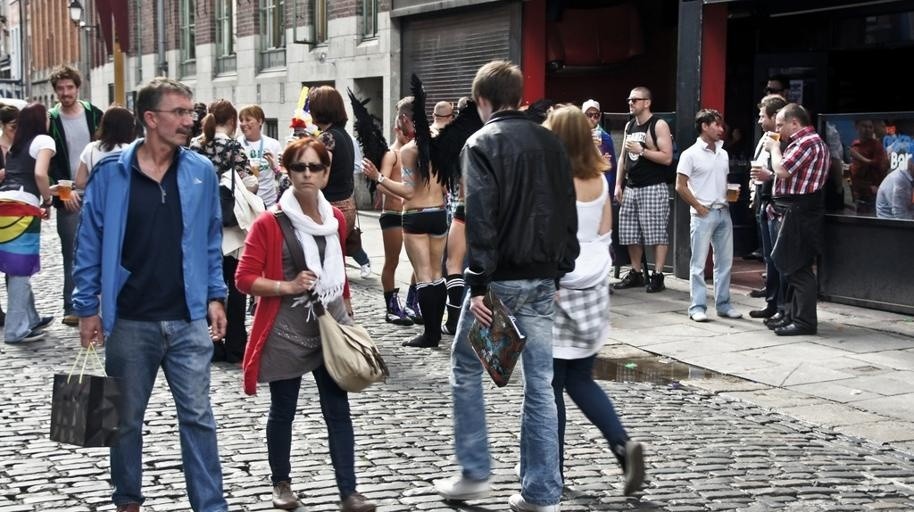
[68,0,100,40]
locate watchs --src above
[376,174,384,185]
[638,147,646,156]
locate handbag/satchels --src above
[217,138,239,227]
[317,309,389,393]
[345,228,363,256]
[50,341,123,447]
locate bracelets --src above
[275,281,280,296]
[43,196,52,208]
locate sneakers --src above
[609,439,645,496]
[726,309,742,318]
[271,480,299,510]
[691,311,709,322]
[613,269,645,289]
[507,494,562,512]
[342,491,376,512]
[647,272,665,293]
[433,477,492,501]
[23,314,79,342]
[361,260,371,278]
[115,503,139,512]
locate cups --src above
[727,184,740,202]
[249,157,260,177]
[57,179,74,201]
[765,131,781,152]
[750,160,763,180]
[592,130,602,147]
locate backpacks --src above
[624,116,682,184]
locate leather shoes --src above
[749,286,816,335]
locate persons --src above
[764,101,833,336]
[233,136,377,512]
[1,65,138,344]
[746,93,789,318]
[824,119,913,221]
[434,64,580,512]
[72,75,228,512]
[751,73,791,281]
[582,98,617,294]
[360,97,484,347]
[613,85,673,293]
[674,108,744,321]
[542,101,643,497]
[185,85,371,364]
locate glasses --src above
[145,108,199,122]
[286,163,326,172]
[625,98,648,103]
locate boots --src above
[384,283,462,347]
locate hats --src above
[582,98,600,114]
